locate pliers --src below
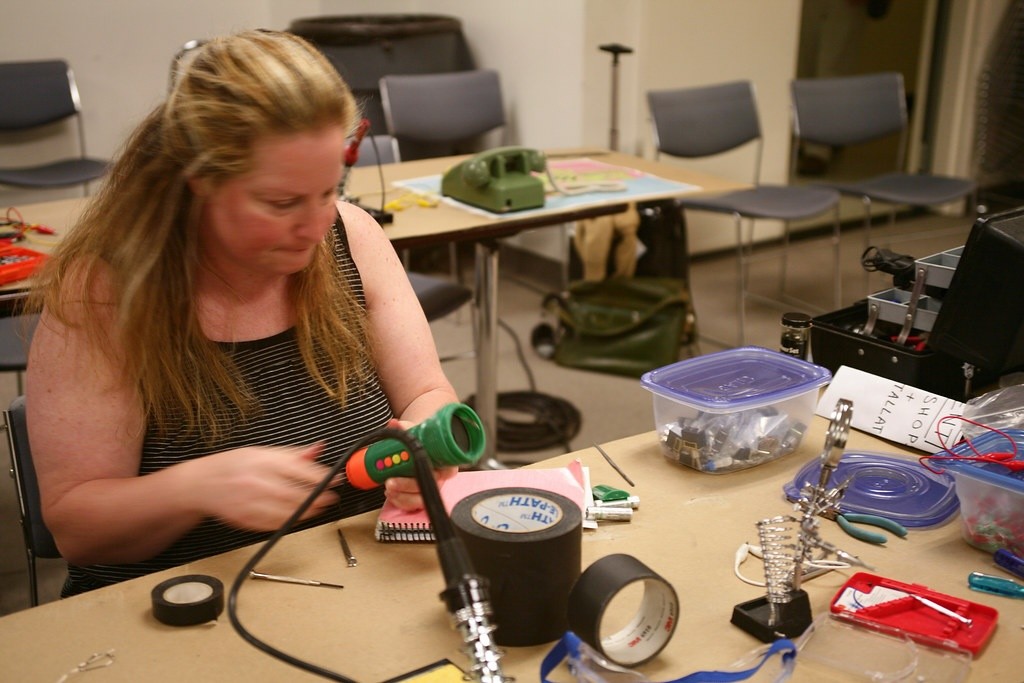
[818,507,908,545]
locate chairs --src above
[776,70,975,313]
[3,404,82,604]
[640,79,843,350]
[0,58,117,200]
[352,134,478,368]
[378,62,569,324]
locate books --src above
[376,461,597,544]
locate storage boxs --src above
[641,345,833,475]
[928,427,1023,558]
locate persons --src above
[26,30,463,596]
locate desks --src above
[0,392,1024,683]
[1,145,755,472]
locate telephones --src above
[442,147,547,214]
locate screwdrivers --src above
[994,549,1023,577]
[969,572,1024,598]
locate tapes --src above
[151,574,224,627]
[565,553,680,668]
[450,488,582,648]
